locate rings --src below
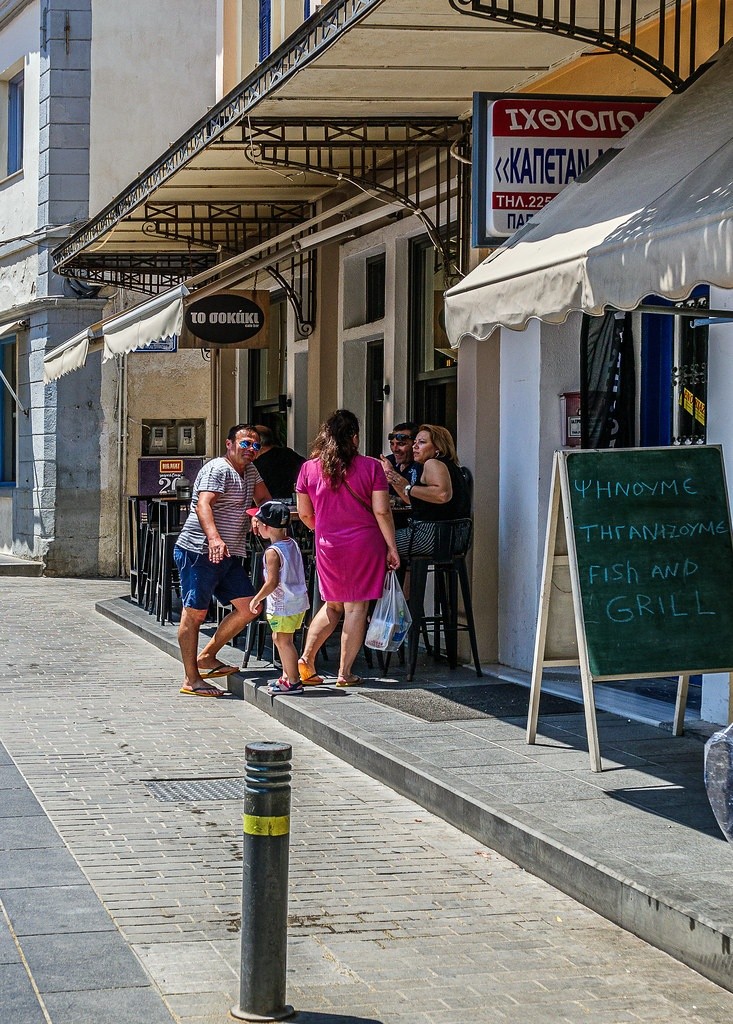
[394,480,396,483]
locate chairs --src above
[127,465,484,682]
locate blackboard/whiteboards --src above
[558,442,733,683]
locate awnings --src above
[43,276,197,390]
[0,309,33,422]
[103,249,249,365]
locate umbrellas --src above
[444,24,733,354]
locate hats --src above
[246,500,291,527]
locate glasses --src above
[234,439,262,450]
[387,433,415,442]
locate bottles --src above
[176,473,191,499]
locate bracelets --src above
[307,525,317,534]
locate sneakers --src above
[266,677,303,696]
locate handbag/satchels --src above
[367,570,414,651]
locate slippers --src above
[199,665,239,678]
[300,674,324,685]
[179,685,224,696]
[336,674,365,687]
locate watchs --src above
[403,480,413,498]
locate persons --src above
[364,423,427,511]
[239,425,311,502]
[246,500,311,694]
[286,408,401,693]
[174,422,285,702]
[370,421,461,582]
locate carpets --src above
[357,682,607,726]
